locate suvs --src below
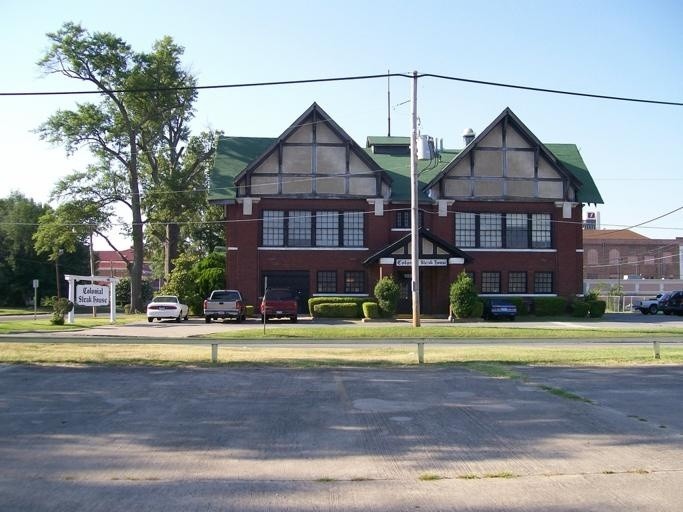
[657,289,683,315]
[259,288,299,321]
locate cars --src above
[146,295,189,322]
[483,299,517,320]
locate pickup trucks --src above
[204,290,247,322]
[631,293,665,316]
